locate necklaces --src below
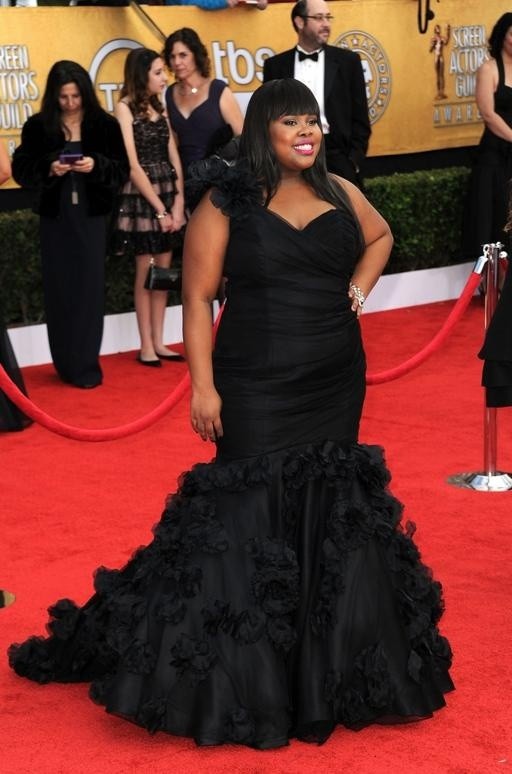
[177,82,208,96]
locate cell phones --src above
[60,154,83,166]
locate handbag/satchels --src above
[144,265,181,290]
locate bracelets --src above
[155,210,168,219]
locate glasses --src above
[303,16,333,21]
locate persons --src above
[159,27,244,324]
[454,10,512,269]
[262,0,372,192]
[11,61,131,387]
[8,79,456,751]
[113,47,186,368]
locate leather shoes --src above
[140,358,161,366]
[157,354,184,362]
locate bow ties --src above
[299,51,318,61]
